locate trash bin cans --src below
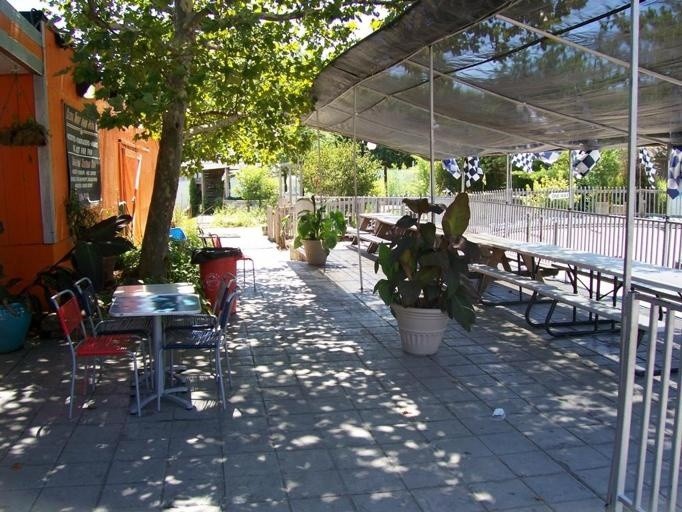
[191,247,242,314]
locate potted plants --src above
[294,195,346,266]
[373,192,482,355]
[0,215,137,353]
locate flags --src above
[535,152,561,166]
[441,160,462,180]
[570,150,601,180]
[639,147,657,190]
[463,157,484,189]
[509,153,534,174]
[665,147,682,223]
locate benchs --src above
[506,256,557,269]
[552,263,681,319]
[469,262,680,375]
[347,227,398,262]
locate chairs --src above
[75,277,151,392]
[209,233,255,291]
[164,285,241,410]
[163,272,238,388]
[199,228,207,247]
[51,289,149,420]
